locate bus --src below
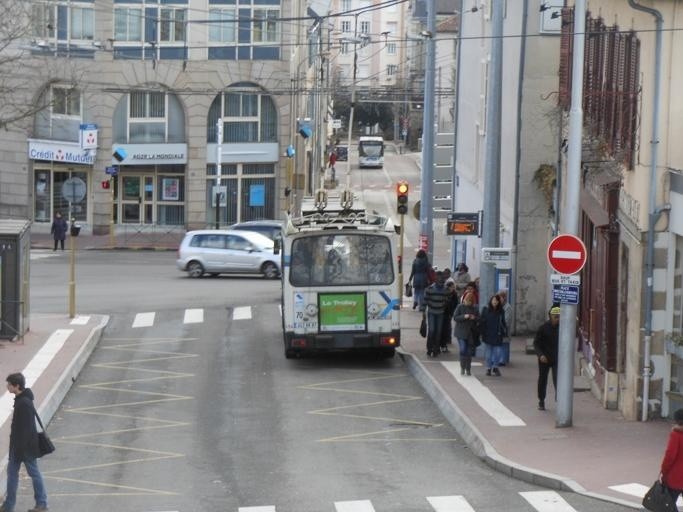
[355,136,386,169]
[272,189,402,365]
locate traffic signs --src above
[551,285,579,306]
[549,273,582,287]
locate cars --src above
[229,219,285,242]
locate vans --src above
[176,228,281,281]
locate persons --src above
[51,211,68,250]
[0,372,50,512]
[534,307,560,410]
[407,249,513,377]
[659,408,683,512]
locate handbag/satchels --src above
[642,480,674,512]
[37,432,54,455]
[420,319,426,336]
[406,282,412,296]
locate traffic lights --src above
[72,224,81,237]
[396,181,409,216]
[410,104,424,109]
[393,223,401,237]
[102,179,110,189]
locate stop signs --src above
[543,232,588,276]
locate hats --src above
[550,307,560,314]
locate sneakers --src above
[487,367,498,375]
[461,368,470,375]
